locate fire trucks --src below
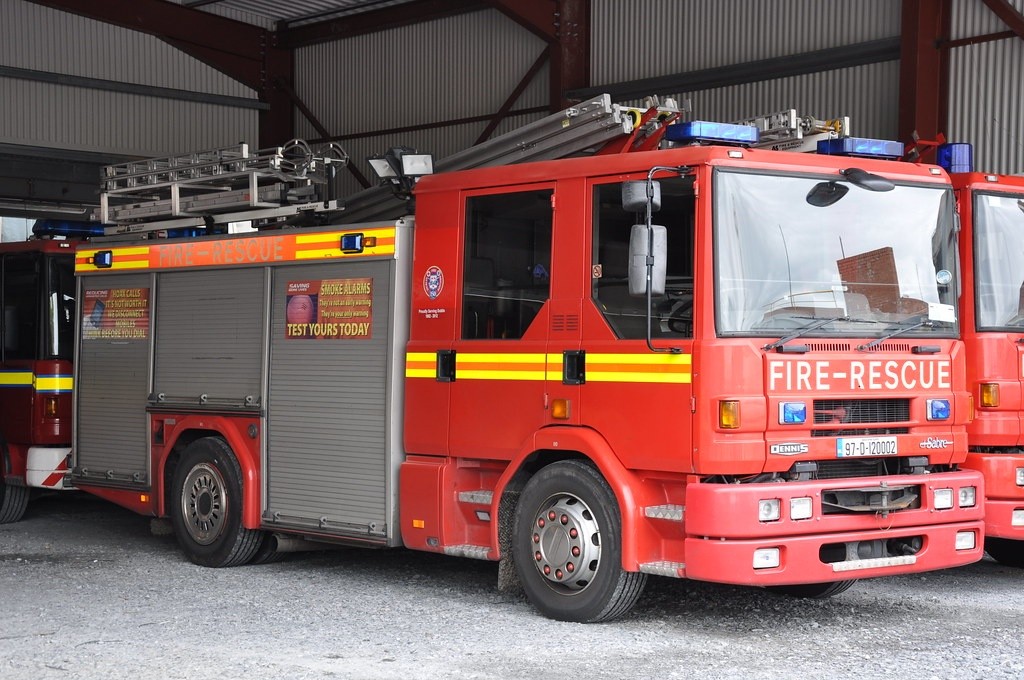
[1,217,227,525]
[936,142,1024,569]
[62,92,986,625]
